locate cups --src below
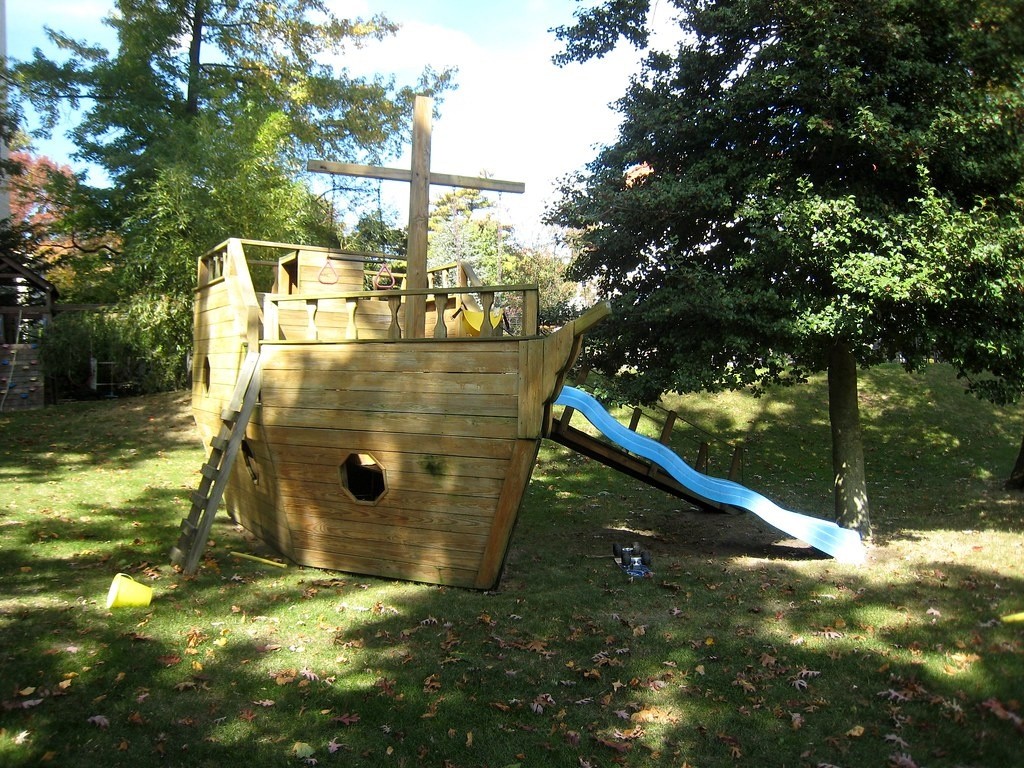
[631,541,641,554]
[621,548,634,568]
[630,557,642,570]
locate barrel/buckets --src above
[106,573,152,609]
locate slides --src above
[552,385,867,561]
